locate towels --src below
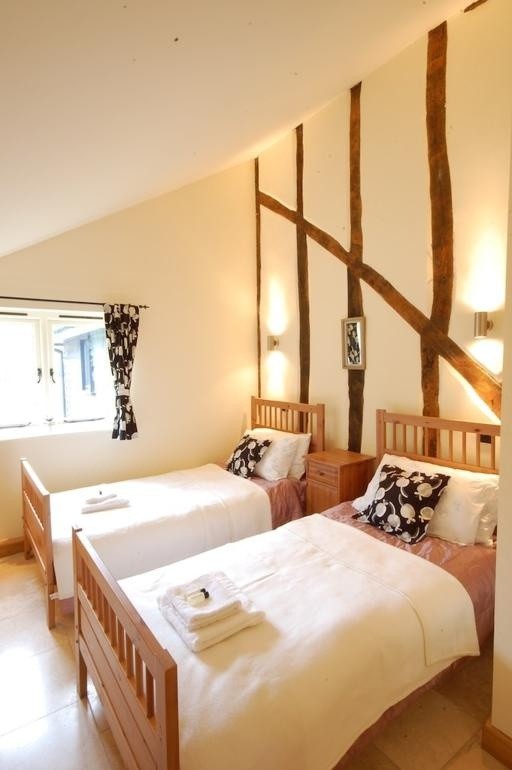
[154,593,265,652]
[82,500,126,513]
[86,491,116,503]
[165,576,241,630]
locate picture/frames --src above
[340,316,366,371]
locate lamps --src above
[475,312,494,339]
[266,336,278,351]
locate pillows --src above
[225,428,312,481]
[352,453,498,544]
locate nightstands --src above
[304,448,376,515]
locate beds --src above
[21,395,326,629]
[71,408,501,770]
